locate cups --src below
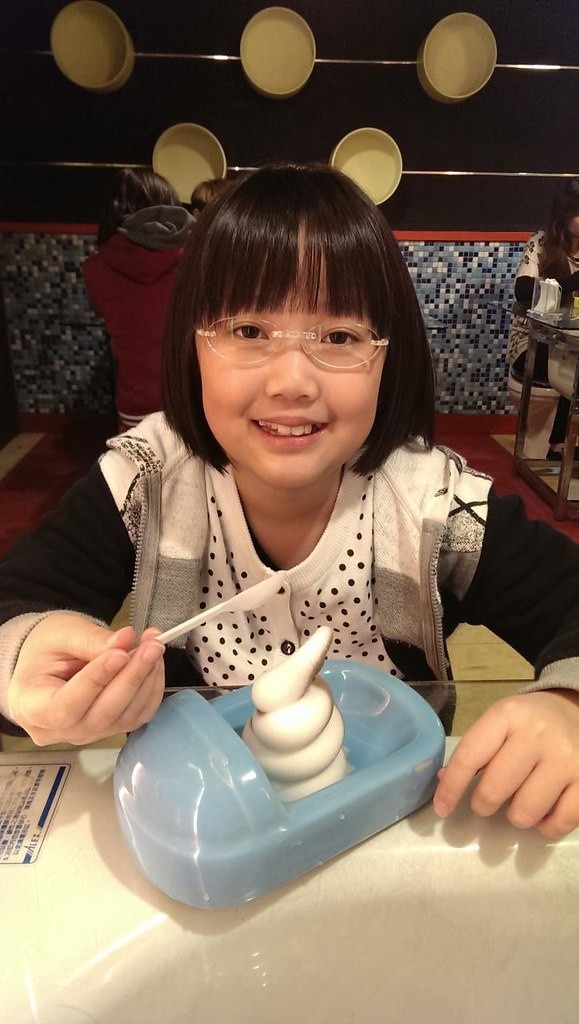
[571,291,579,317]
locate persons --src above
[80,165,200,438]
[191,179,237,221]
[0,164,579,841]
[506,176,579,462]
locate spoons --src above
[127,570,286,653]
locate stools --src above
[505,368,562,460]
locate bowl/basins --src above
[152,123,226,206]
[115,659,445,910]
[51,1,134,93]
[328,127,402,206]
[241,6,316,97]
[416,13,497,103]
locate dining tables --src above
[0,673,579,1024]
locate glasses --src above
[195,315,390,370]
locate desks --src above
[486,301,579,523]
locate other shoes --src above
[545,444,579,462]
